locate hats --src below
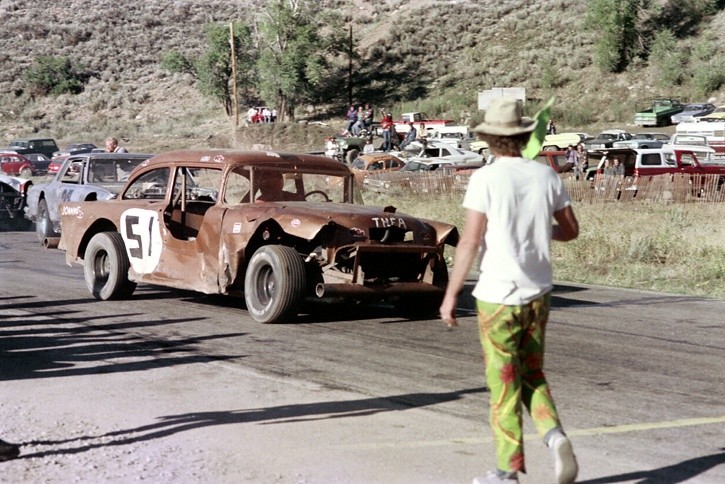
[468,96,538,136]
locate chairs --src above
[82,166,105,183]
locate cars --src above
[245,107,272,123]
[533,103,725,187]
[325,112,494,190]
[0,153,218,246]
[44,149,459,324]
[0,138,98,177]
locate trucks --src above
[635,99,684,127]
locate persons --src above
[247,105,277,124]
[557,144,588,180]
[392,122,418,151]
[603,158,625,177]
[380,113,393,153]
[416,123,428,150]
[342,103,373,136]
[439,99,578,484]
[105,138,129,154]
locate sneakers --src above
[548,430,578,484]
[473,470,519,484]
